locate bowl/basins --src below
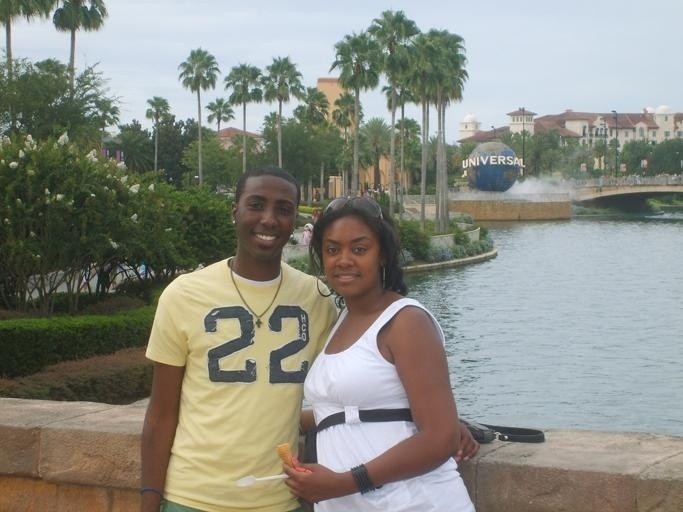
[229,257,283,329]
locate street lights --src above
[460,416,496,442]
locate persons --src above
[139,162,483,512]
[284,196,478,512]
[301,227,312,248]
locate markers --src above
[276,443,294,469]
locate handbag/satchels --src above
[140,487,164,499]
[351,463,375,495]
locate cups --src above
[324,196,384,221]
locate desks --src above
[491,126,496,139]
[611,110,619,186]
[519,107,526,184]
